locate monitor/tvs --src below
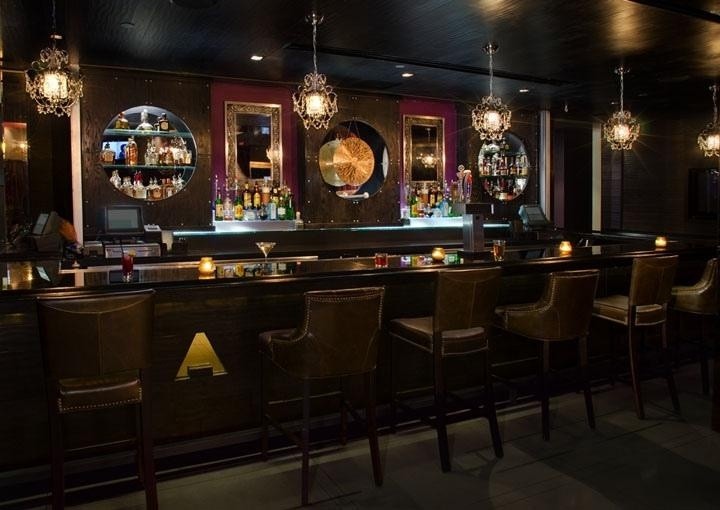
[31,211,56,235]
[518,204,549,225]
[101,203,145,236]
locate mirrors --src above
[403,114,446,190]
[226,102,281,186]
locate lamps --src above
[292,13,338,131]
[473,43,512,139]
[25,2,82,117]
[601,68,639,149]
[422,127,439,168]
[697,85,720,156]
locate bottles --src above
[445,194,450,206]
[163,150,168,166]
[505,143,509,156]
[116,112,129,129]
[435,182,443,202]
[130,142,138,165]
[519,159,522,175]
[291,193,296,220]
[268,193,276,220]
[172,175,177,193]
[286,192,295,220]
[428,184,432,203]
[186,152,191,166]
[486,156,491,175]
[505,157,509,176]
[145,140,151,165]
[293,212,303,230]
[125,138,133,165]
[215,188,223,221]
[499,187,507,201]
[262,177,270,206]
[154,117,162,131]
[223,177,232,201]
[235,189,243,221]
[119,177,126,192]
[434,203,442,217]
[483,160,489,175]
[176,173,185,191]
[430,190,436,208]
[182,146,187,166]
[223,189,233,220]
[253,185,260,210]
[150,147,158,166]
[242,181,252,210]
[507,175,514,192]
[284,186,288,199]
[493,186,500,199]
[124,176,133,197]
[179,153,184,166]
[416,186,421,202]
[114,170,121,189]
[440,198,449,217]
[110,171,115,184]
[491,156,496,176]
[277,197,286,220]
[522,157,527,175]
[133,170,138,198]
[166,149,173,166]
[280,186,285,199]
[500,137,506,156]
[410,192,417,217]
[489,181,493,197]
[151,177,162,199]
[421,182,428,203]
[484,178,489,191]
[135,109,154,130]
[278,188,282,199]
[160,178,166,198]
[134,170,147,199]
[232,178,242,203]
[513,177,521,197]
[165,178,176,199]
[285,200,288,220]
[448,193,454,217]
[175,151,179,166]
[498,160,507,176]
[509,157,516,175]
[508,183,513,200]
[146,177,153,200]
[517,162,519,175]
[245,203,256,221]
[100,144,116,165]
[501,178,506,188]
[159,113,170,131]
[212,201,215,221]
[158,151,164,166]
[424,203,433,218]
[497,179,501,189]
[417,194,425,217]
[270,189,280,209]
[259,202,268,220]
[415,182,419,196]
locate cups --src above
[255,241,276,263]
[493,240,506,260]
[121,254,135,283]
[374,252,388,269]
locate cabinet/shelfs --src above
[478,131,531,203]
[1,229,706,510]
[100,105,198,202]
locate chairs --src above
[672,259,720,428]
[388,266,504,472]
[257,285,385,509]
[593,253,683,421]
[494,269,600,440]
[33,289,158,509]
